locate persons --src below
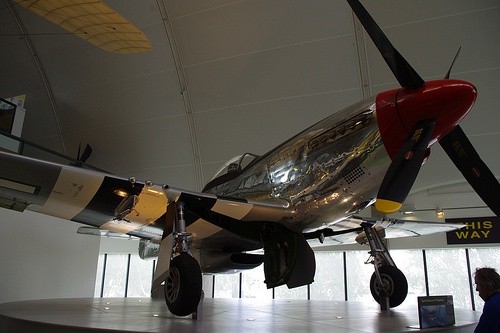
[472,267,500,333]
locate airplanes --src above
[0,0,500,317]
